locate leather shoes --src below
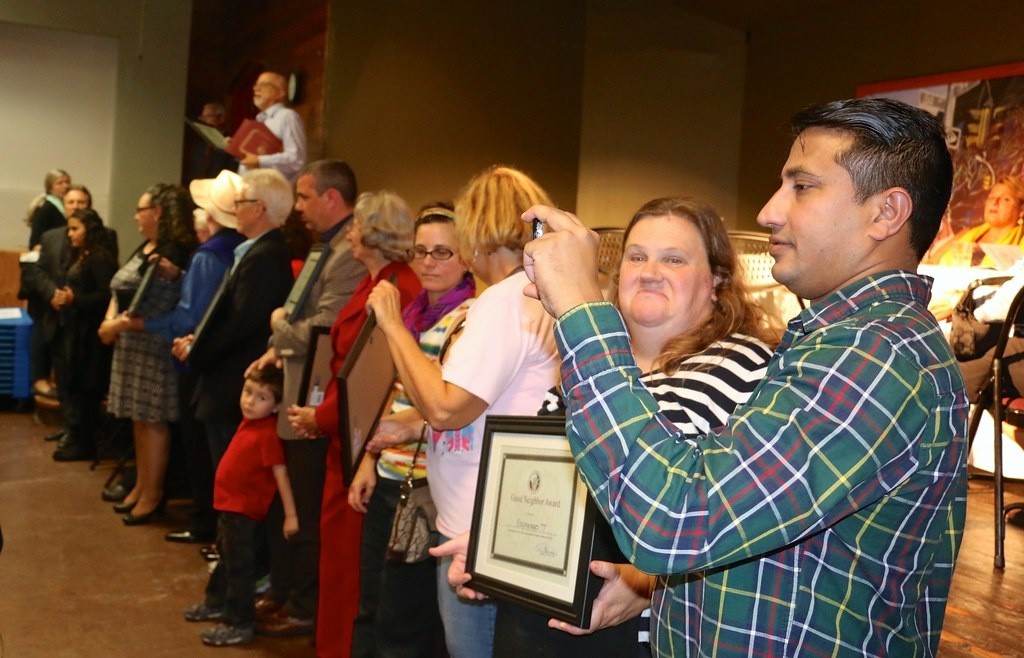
[252,590,279,613]
[252,611,317,636]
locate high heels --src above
[122,488,169,523]
[114,499,137,514]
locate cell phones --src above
[532,218,544,239]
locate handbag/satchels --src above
[390,487,442,571]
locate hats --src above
[191,169,246,234]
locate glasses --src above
[134,204,155,215]
[406,245,458,260]
[231,197,258,208]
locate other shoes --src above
[56,431,75,447]
[165,530,200,543]
[200,546,215,554]
[203,551,220,560]
[102,485,129,500]
[45,428,68,442]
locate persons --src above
[163,168,249,543]
[50,209,117,461]
[288,190,429,657]
[430,197,775,658]
[192,101,236,179]
[367,163,565,658]
[193,207,211,244]
[235,72,308,196]
[99,181,189,527]
[37,186,119,398]
[17,169,74,400]
[946,282,1024,403]
[348,200,484,657]
[926,174,1024,268]
[242,159,371,636]
[520,98,971,658]
[123,168,295,561]
[184,361,300,645]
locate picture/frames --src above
[855,62,1024,280]
[464,412,630,636]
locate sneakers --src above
[201,622,254,647]
[185,603,229,621]
